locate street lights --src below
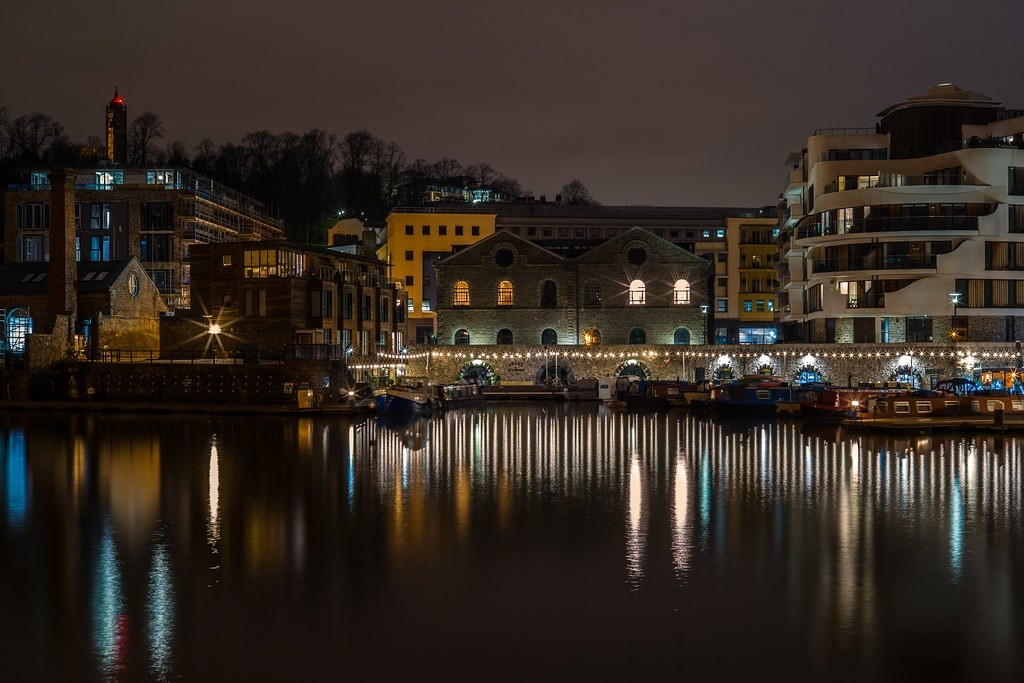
[700,305,709,345]
[948,293,963,342]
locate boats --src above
[367,379,481,423]
[562,374,1024,429]
